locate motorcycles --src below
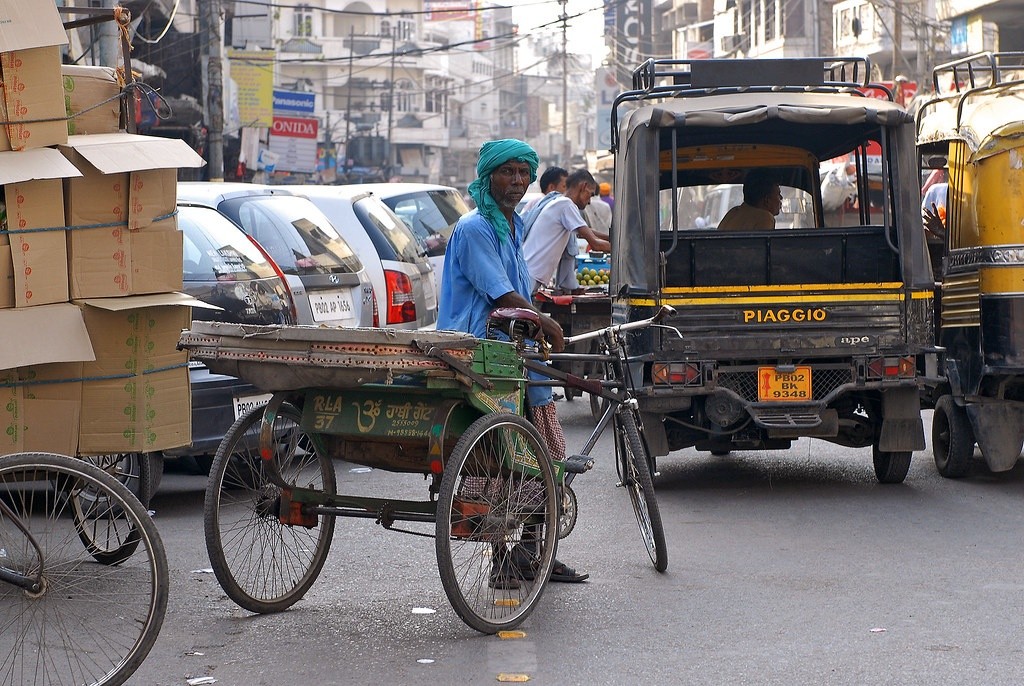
[913,51,1024,481]
[609,56,947,486]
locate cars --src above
[240,184,437,332]
[341,181,474,312]
[515,193,544,214]
[0,201,301,518]
[173,181,379,331]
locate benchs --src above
[657,226,897,285]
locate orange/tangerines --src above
[576,267,610,286]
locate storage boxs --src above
[0,0,226,460]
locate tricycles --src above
[52,447,152,566]
[204,304,685,637]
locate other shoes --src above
[552,392,564,401]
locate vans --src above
[696,182,796,230]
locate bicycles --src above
[0,452,169,686]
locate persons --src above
[436,141,589,588]
[521,166,784,405]
[923,202,946,240]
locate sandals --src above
[489,556,519,589]
[512,545,589,583]
